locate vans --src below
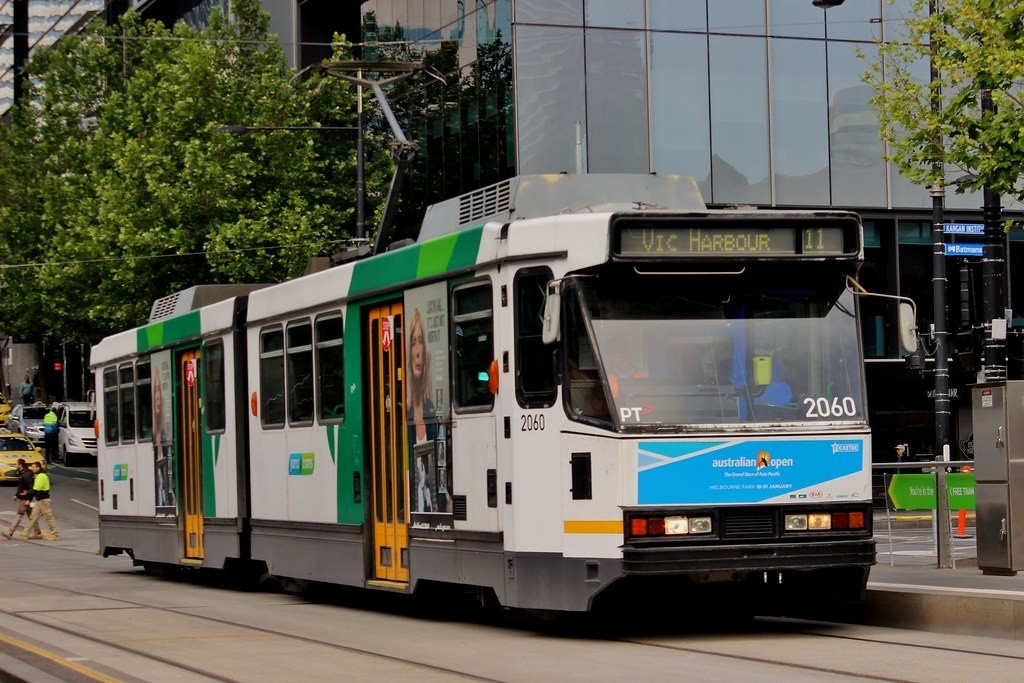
[51,401,98,467]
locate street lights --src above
[216,54,450,247]
[813,1,949,456]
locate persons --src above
[153,368,174,515]
[598,340,656,423]
[6,415,18,433]
[43,407,59,463]
[17,461,60,541]
[2,459,43,540]
[19,375,37,407]
[407,298,447,508]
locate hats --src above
[895,444,905,448]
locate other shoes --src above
[28,534,43,539]
[15,531,29,540]
[46,534,61,541]
[1,530,14,541]
[46,459,51,464]
[54,459,61,463]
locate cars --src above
[0,393,14,426]
[0,427,48,486]
[5,401,50,445]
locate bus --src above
[88,173,917,626]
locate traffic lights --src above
[53,350,62,370]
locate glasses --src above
[897,449,903,450]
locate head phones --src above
[16,469,26,476]
